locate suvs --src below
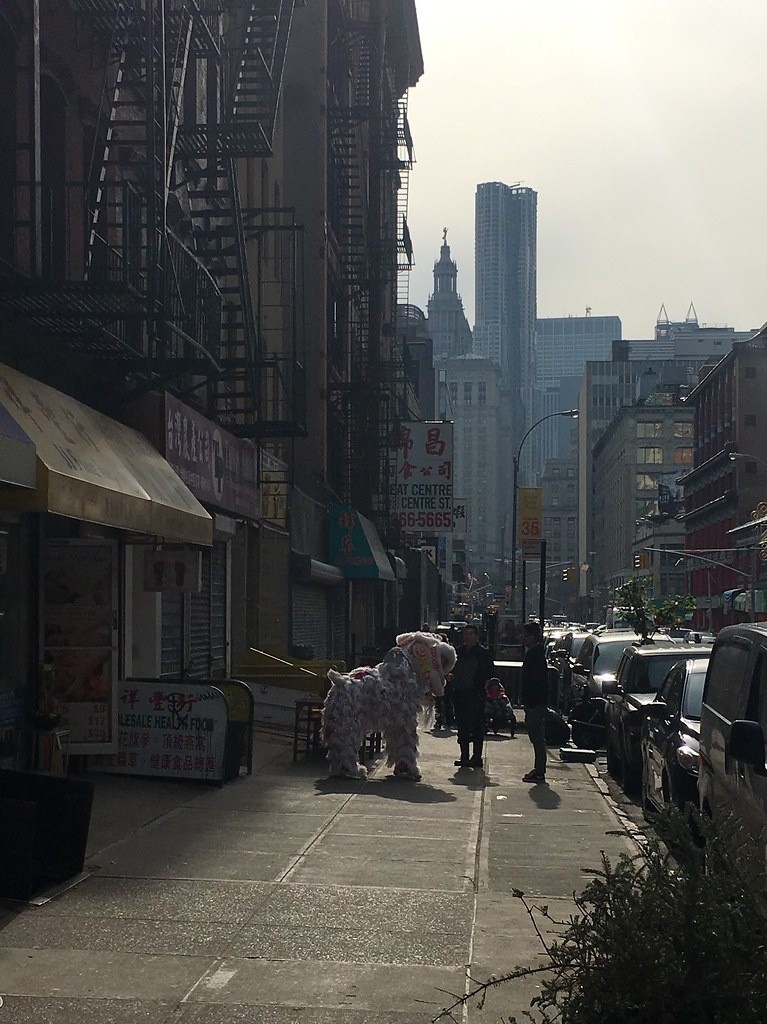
[570,632,675,745]
[600,634,713,793]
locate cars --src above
[636,659,709,844]
[530,615,713,732]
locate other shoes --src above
[464,755,483,767]
[522,769,544,783]
[454,760,469,765]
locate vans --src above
[699,621,766,921]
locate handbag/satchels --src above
[543,709,570,746]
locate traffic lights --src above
[562,568,568,581]
[507,580,511,585]
[633,555,641,568]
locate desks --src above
[293,697,325,763]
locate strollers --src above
[484,707,516,737]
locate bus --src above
[606,607,635,631]
[551,615,567,627]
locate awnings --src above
[0,361,213,547]
[329,504,394,581]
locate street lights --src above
[511,406,580,607]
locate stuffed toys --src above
[319,630,456,781]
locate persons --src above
[518,622,550,782]
[433,633,454,729]
[385,620,396,649]
[454,623,494,768]
[446,624,456,642]
[422,623,431,632]
